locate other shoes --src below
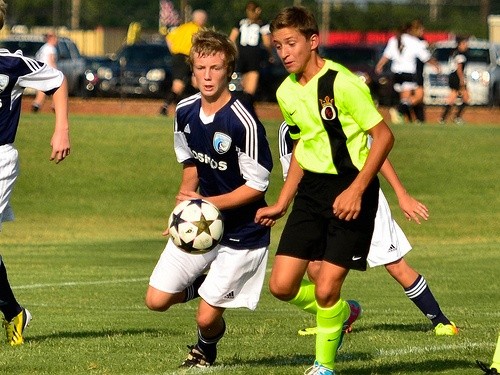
[388,105,406,125]
[31,101,42,114]
[158,105,169,118]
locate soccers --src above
[167,198,225,255]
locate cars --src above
[422,41,500,106]
[0,33,87,96]
[322,42,401,104]
[95,40,184,99]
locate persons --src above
[0,0,71,350]
[144,0,500,375]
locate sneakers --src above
[303,361,337,375]
[434,320,459,337]
[0,307,33,346]
[178,344,217,371]
[336,299,364,356]
